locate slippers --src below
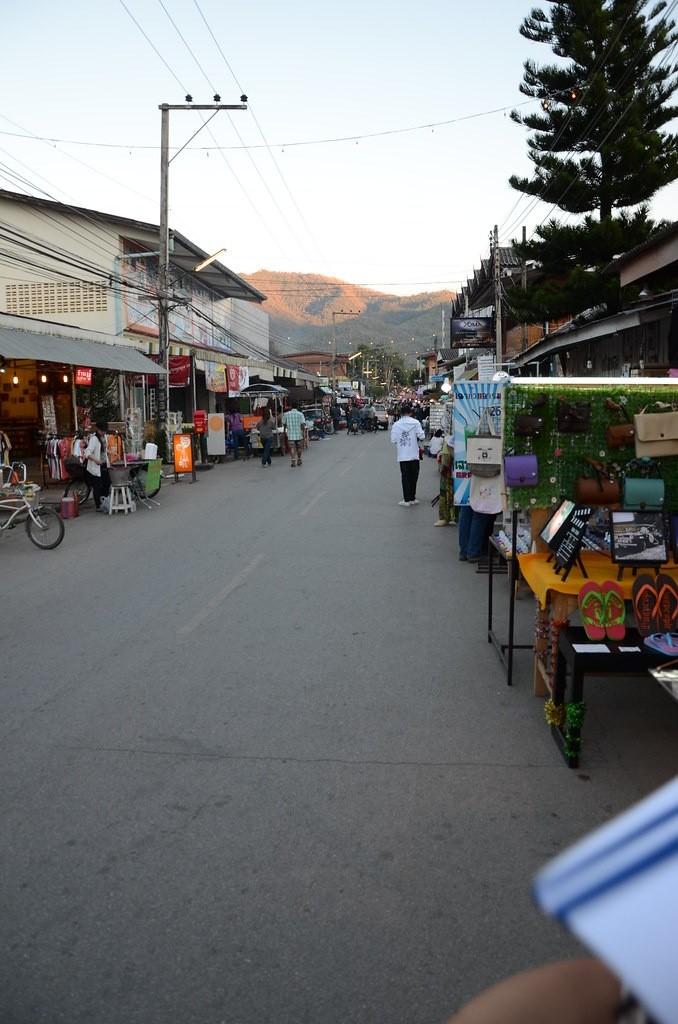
[577,581,606,641]
[601,580,626,641]
[632,573,659,637]
[656,574,678,634]
[644,632,678,656]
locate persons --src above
[430,429,444,456]
[452,776,678,1024]
[435,436,458,526]
[391,404,425,507]
[84,420,118,512]
[257,409,276,468]
[331,400,430,436]
[458,506,498,563]
[282,403,306,467]
[227,408,250,460]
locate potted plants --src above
[80,368,126,433]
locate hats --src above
[95,419,110,431]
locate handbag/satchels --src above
[513,404,543,438]
[60,490,79,519]
[557,401,590,433]
[605,405,637,449]
[621,462,665,513]
[505,454,538,488]
[466,408,502,477]
[469,474,502,514]
[633,402,678,459]
[573,465,620,512]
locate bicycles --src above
[64,455,164,505]
[0,462,65,550]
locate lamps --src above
[639,286,654,300]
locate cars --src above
[392,398,398,405]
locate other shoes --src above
[95,509,102,512]
[262,464,267,468]
[409,499,419,505]
[269,459,271,465]
[297,461,302,466]
[448,520,458,526]
[398,499,411,507]
[434,519,447,526]
[291,463,296,467]
[458,555,488,563]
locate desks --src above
[551,626,678,769]
[113,458,163,508]
[516,550,678,700]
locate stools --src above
[109,486,134,515]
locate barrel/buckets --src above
[110,470,130,485]
[61,497,79,518]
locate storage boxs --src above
[628,364,669,377]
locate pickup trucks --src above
[363,404,389,430]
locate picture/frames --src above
[539,498,577,545]
[610,510,668,564]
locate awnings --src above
[127,332,323,383]
[0,327,171,375]
[506,298,678,368]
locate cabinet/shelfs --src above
[0,419,38,459]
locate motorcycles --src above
[303,410,335,441]
[351,421,359,434]
